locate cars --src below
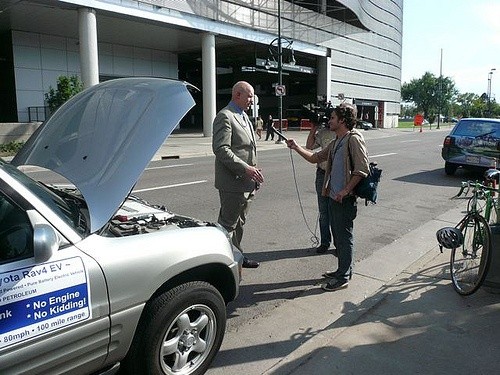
[444,117,457,123]
[441,117,500,179]
[0,75,244,375]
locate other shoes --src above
[242,257,259,268]
[320,276,349,291]
[324,269,353,280]
[316,244,329,254]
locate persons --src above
[255,115,264,139]
[285,102,369,293]
[212,80,264,269]
[306,103,343,254]
[264,114,274,141]
[364,110,369,122]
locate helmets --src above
[436,227,465,250]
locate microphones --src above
[269,115,274,129]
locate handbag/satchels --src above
[352,162,383,207]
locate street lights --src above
[487,68,496,115]
[264,35,294,144]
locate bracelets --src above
[344,185,353,195]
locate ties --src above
[242,114,248,126]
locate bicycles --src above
[437,168,499,296]
